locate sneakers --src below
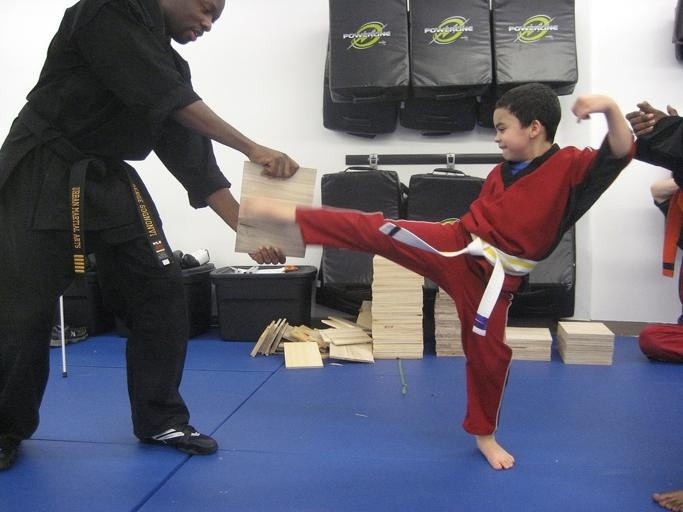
[136,423,220,458]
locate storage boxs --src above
[181,263,216,341]
[209,265,318,342]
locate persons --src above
[639,178,683,366]
[1,1,301,480]
[624,96,683,512]
[233,82,635,471]
